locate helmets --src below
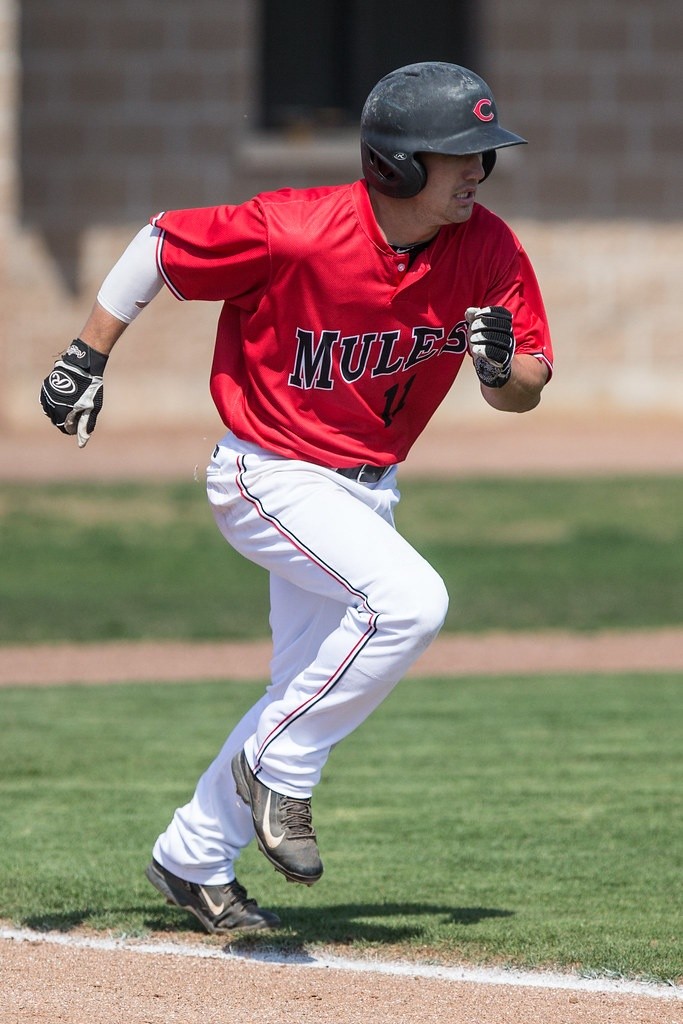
[360,61,528,199]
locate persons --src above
[39,62,554,936]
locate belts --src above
[335,464,389,484]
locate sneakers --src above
[231,747,323,888]
[146,856,281,936]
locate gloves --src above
[465,305,515,390]
[39,338,110,448]
